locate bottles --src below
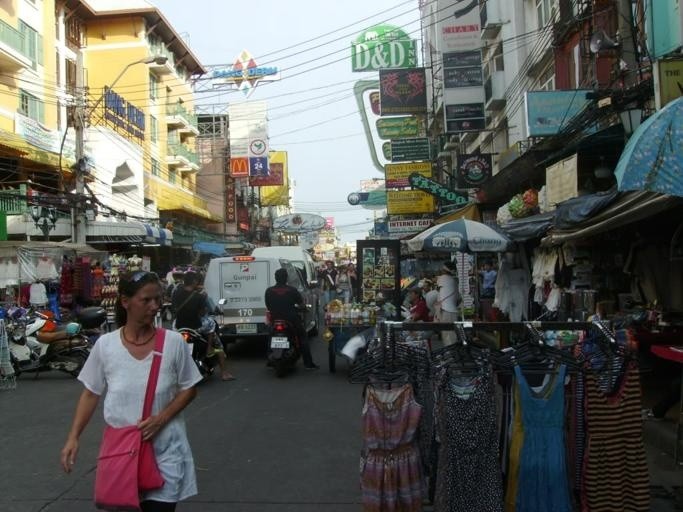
[99,268,118,307]
[564,317,580,344]
[325,297,376,325]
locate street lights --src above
[26,190,58,242]
[60,56,173,251]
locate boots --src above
[302,353,320,369]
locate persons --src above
[166,258,498,381]
[59,267,206,511]
[128,254,142,271]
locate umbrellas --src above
[407,215,520,304]
[612,95,682,199]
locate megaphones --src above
[591,29,620,54]
[72,159,85,173]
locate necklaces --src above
[121,324,157,347]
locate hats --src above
[405,286,423,297]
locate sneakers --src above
[221,371,237,381]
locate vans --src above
[198,245,321,346]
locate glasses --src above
[126,270,161,286]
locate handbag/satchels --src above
[92,325,168,509]
[432,301,442,320]
[265,307,273,327]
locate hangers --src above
[344,323,639,384]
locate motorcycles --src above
[172,297,234,388]
[3,292,110,379]
[259,301,314,377]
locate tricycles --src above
[323,325,381,374]
